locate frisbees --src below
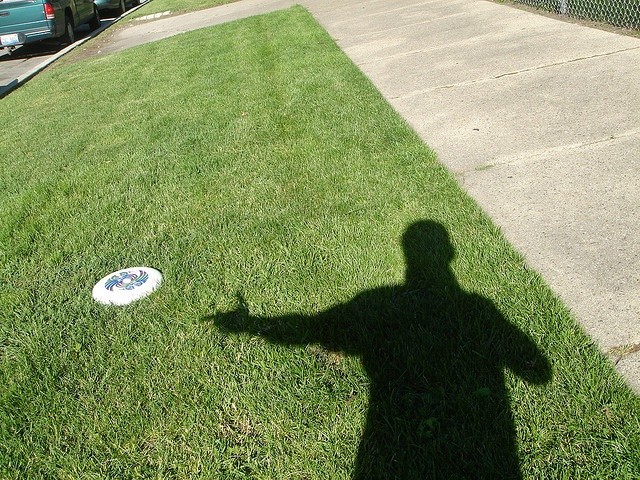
[92,267,162,306]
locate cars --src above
[96,0,141,18]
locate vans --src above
[0,0,101,49]
